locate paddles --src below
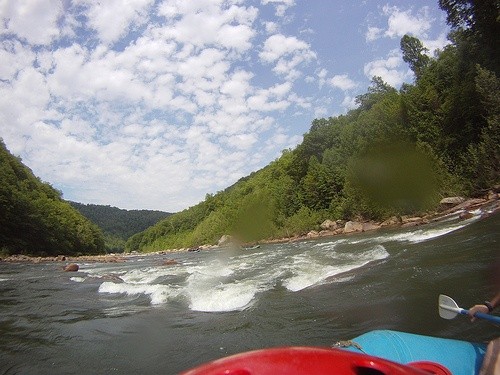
[439,295,499,325]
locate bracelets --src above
[484,301,494,312]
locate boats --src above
[173,328,487,375]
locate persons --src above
[467,290,500,375]
